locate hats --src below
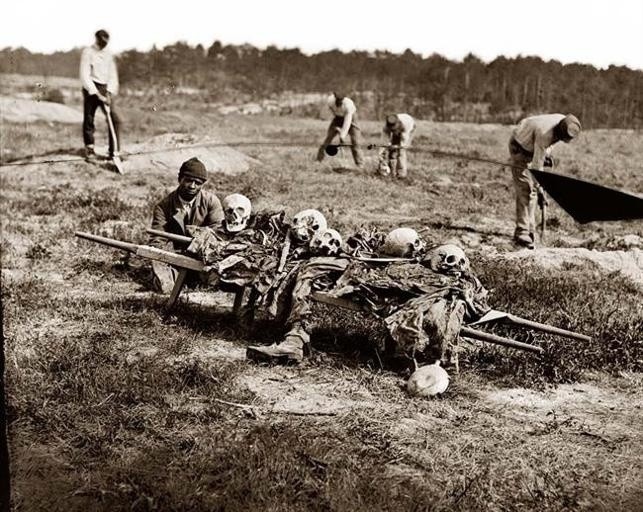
[560,113,582,144]
[334,90,345,101]
[179,156,208,182]
[386,113,400,130]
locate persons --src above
[80,29,124,162]
[374,112,416,178]
[508,113,581,249]
[316,90,366,169]
[148,157,224,295]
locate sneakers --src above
[513,229,537,251]
[108,152,123,162]
[245,341,304,365]
[85,145,96,159]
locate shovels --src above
[104,103,125,174]
